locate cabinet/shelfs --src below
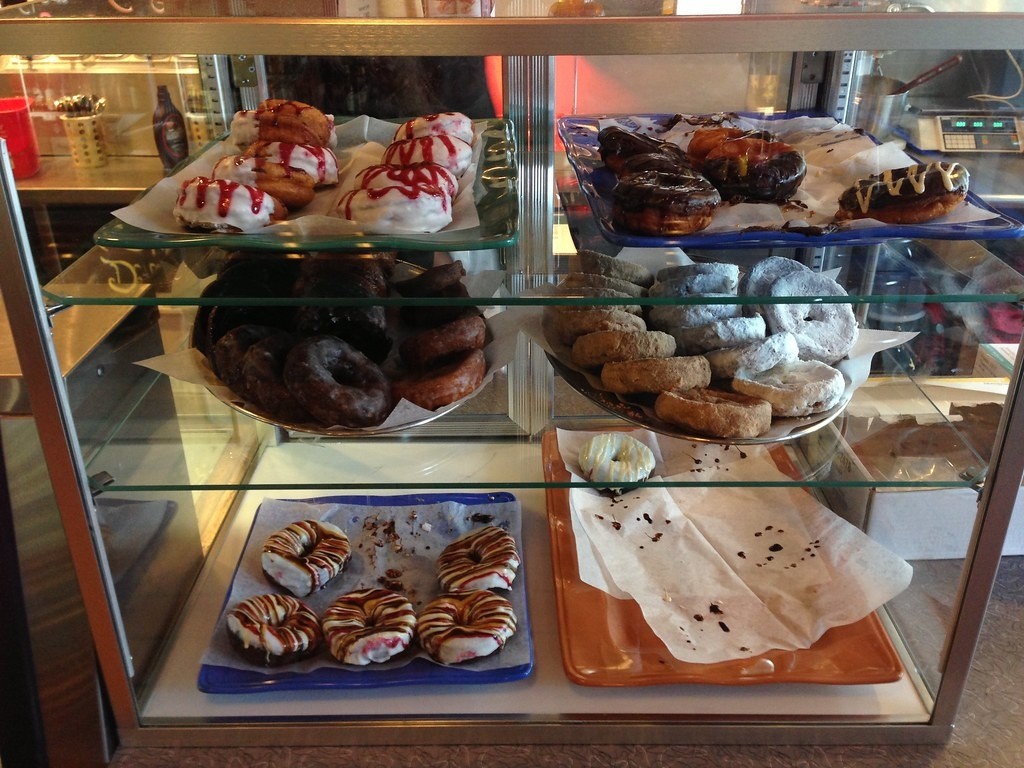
[0,0,1024,748]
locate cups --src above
[855,75,907,137]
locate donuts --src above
[207,251,488,428]
[550,250,858,439]
[598,112,971,239]
[224,519,520,664]
[173,99,474,235]
[578,432,655,496]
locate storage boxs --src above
[804,419,1024,561]
[972,342,1020,380]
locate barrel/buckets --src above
[0,97,40,179]
[847,288,926,370]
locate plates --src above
[93,112,521,250]
[544,256,854,445]
[187,252,493,438]
[559,112,1023,248]
[543,425,902,686]
[195,491,533,694]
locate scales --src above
[895,94,1024,154]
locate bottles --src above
[153,85,189,170]
